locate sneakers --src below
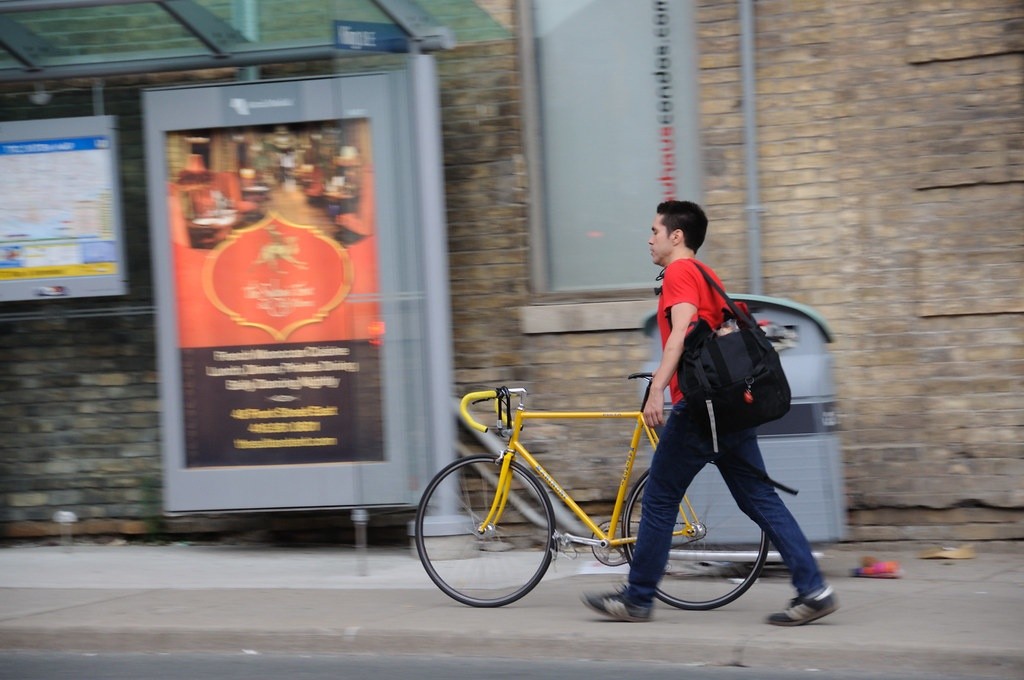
[767,586,840,626]
[580,584,651,623]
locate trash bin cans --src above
[636,292,847,573]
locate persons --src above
[578,199,839,627]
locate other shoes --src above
[852,563,904,578]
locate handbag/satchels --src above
[655,261,792,435]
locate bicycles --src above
[415,372,770,608]
